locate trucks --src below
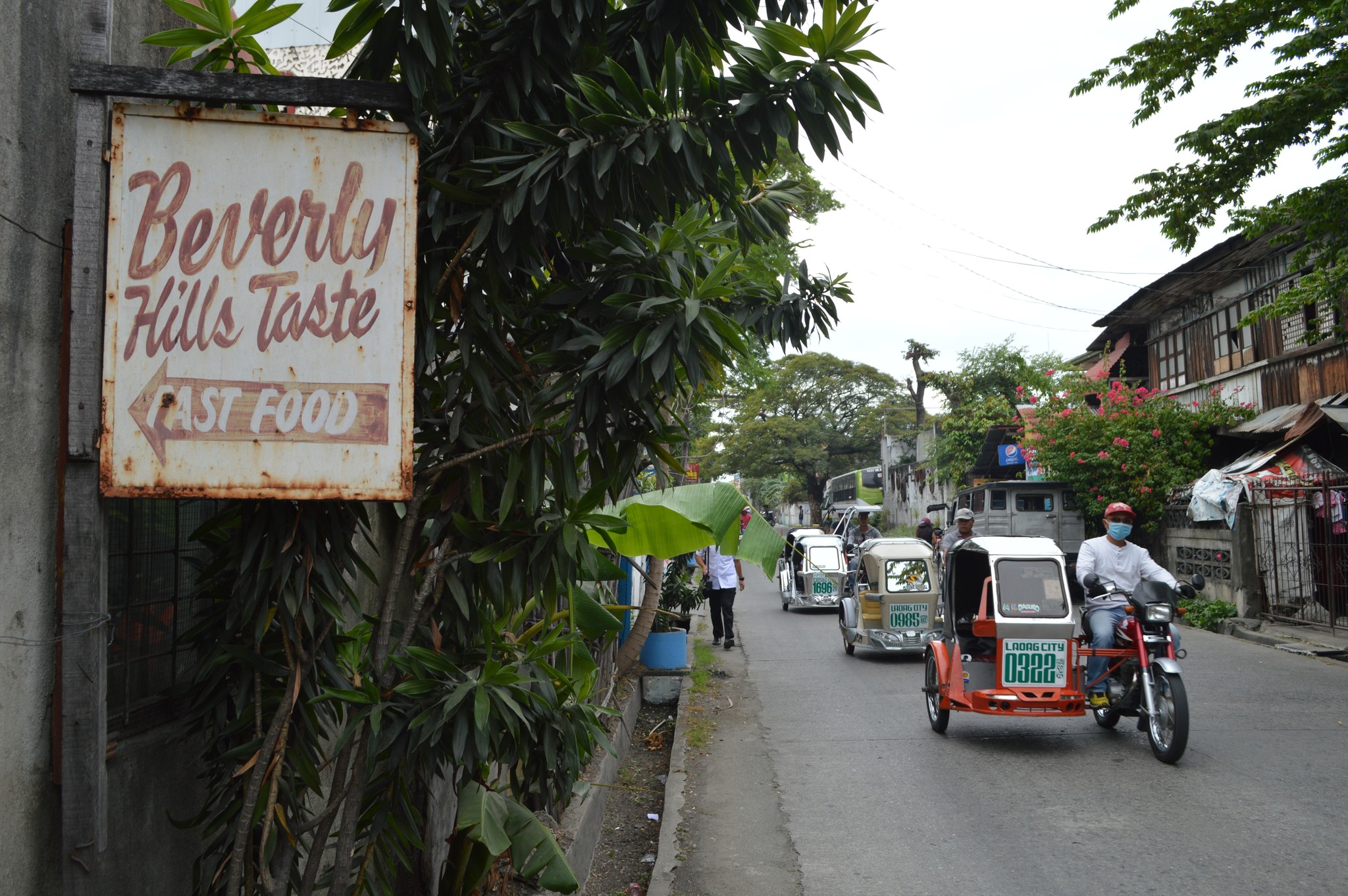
[926,479,1087,590]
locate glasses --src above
[1106,517,1131,524]
[862,533,866,542]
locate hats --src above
[917,517,931,527]
[1104,502,1136,519]
[955,508,974,520]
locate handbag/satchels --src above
[698,574,712,599]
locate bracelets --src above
[739,577,744,581]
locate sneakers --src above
[1088,691,1111,708]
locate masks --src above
[1104,519,1133,540]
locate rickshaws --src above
[919,536,1208,764]
[838,537,944,655]
[739,505,883,611]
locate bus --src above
[824,465,883,518]
[821,490,832,523]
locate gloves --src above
[1089,583,1107,600]
[846,544,854,552]
[1179,585,1196,599]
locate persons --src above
[694,544,745,650]
[829,513,857,542]
[761,505,770,519]
[942,507,984,569]
[740,508,752,536]
[796,505,804,527]
[846,511,883,596]
[915,518,937,547]
[1075,501,1198,711]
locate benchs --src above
[962,651,999,692]
[859,590,882,620]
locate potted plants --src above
[640,551,710,672]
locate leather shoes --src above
[724,637,734,649]
[713,637,721,645]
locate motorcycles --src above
[931,528,946,561]
